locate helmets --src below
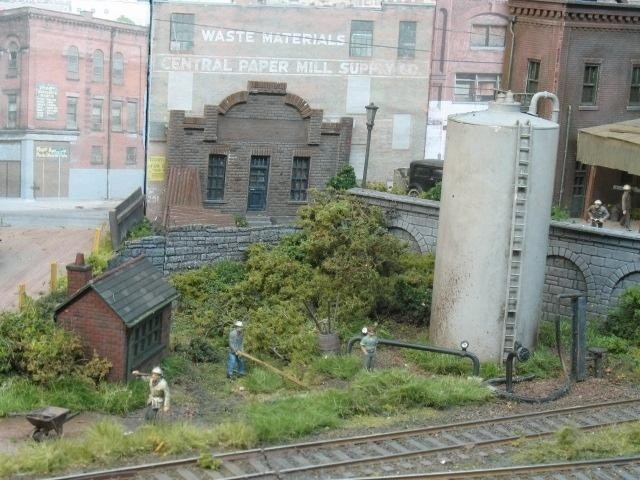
[151,366,164,376]
[623,184,631,190]
[594,199,602,204]
[233,320,245,328]
